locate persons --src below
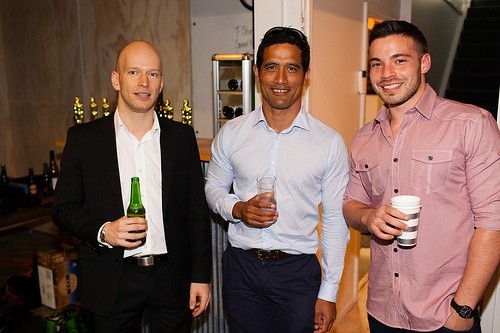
[342,19,500,333]
[203,26,351,333]
[49,40,213,333]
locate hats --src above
[263,27,306,44]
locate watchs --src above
[450,298,478,319]
[100,228,105,243]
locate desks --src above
[0,174,60,235]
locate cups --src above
[390,194,422,247]
[228,79,242,91]
[256,174,277,224]
[222,104,243,119]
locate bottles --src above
[0,163,10,198]
[42,162,50,196]
[48,150,59,198]
[127,176,146,246]
[27,167,39,208]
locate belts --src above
[247,248,291,261]
[123,254,168,267]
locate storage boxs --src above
[38,244,80,311]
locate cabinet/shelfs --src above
[211,53,255,139]
